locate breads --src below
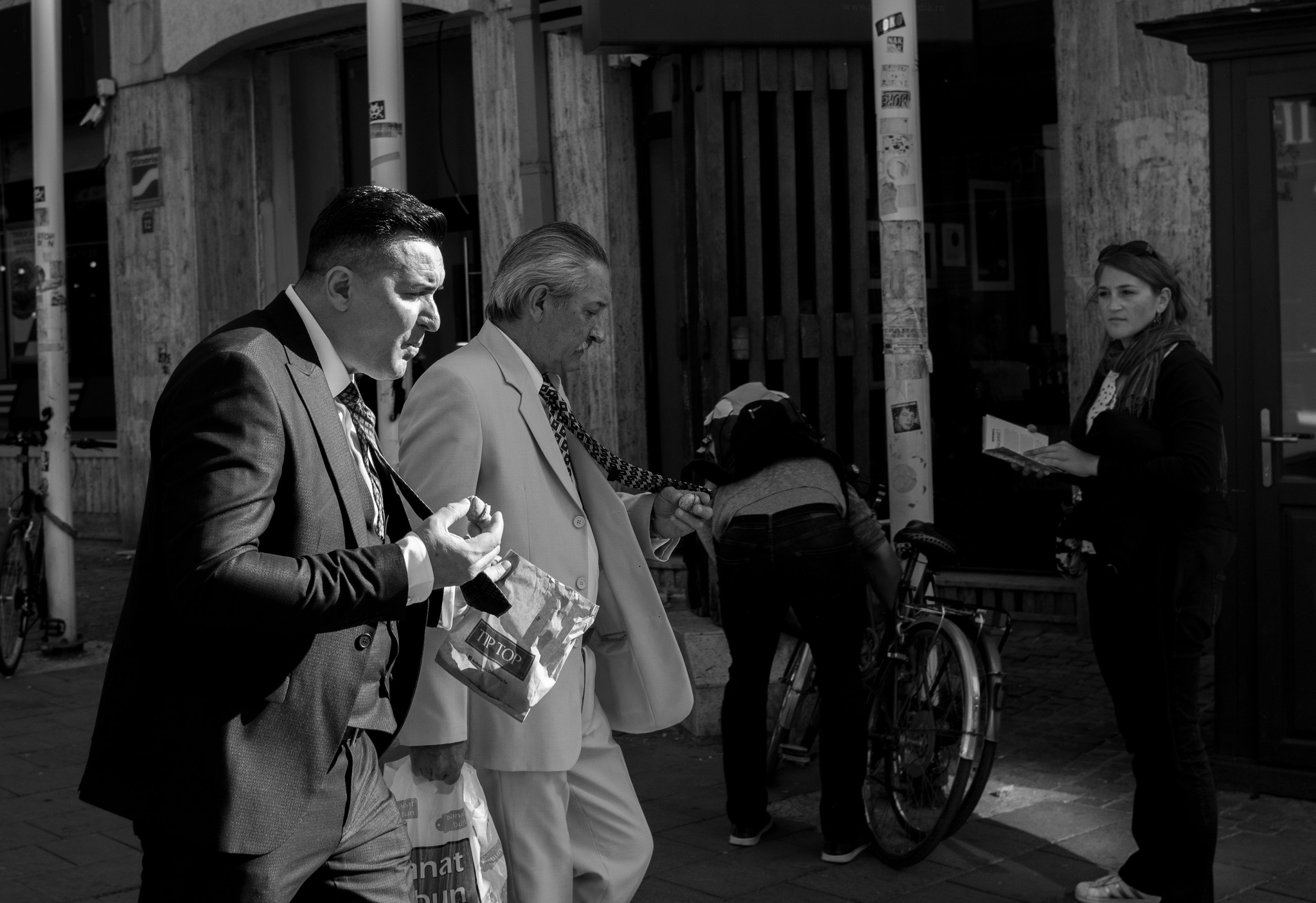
[678,493,701,513]
[465,496,491,523]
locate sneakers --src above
[1075,872,1162,903]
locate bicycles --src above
[0,407,76,677]
[768,459,1013,870]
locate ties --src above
[536,382,711,498]
[337,384,512,629]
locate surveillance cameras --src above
[80,104,105,128]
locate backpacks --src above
[696,380,848,501]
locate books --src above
[982,416,1064,475]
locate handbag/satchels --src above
[381,756,508,903]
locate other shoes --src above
[727,810,773,845]
[821,834,866,862]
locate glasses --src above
[1098,239,1156,259]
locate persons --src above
[396,221,713,903]
[1011,238,1228,903]
[78,185,511,903]
[694,384,903,863]
